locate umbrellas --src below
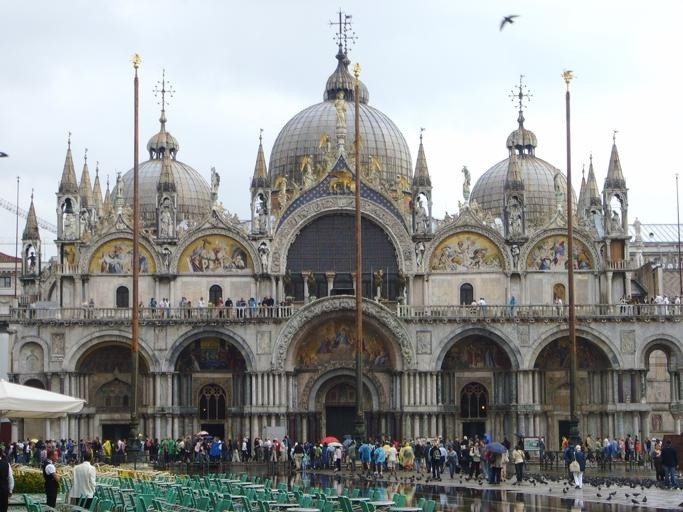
[0,375,89,421]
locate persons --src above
[185,235,246,272]
[0,447,14,512]
[297,324,389,367]
[569,444,586,489]
[416,200,428,235]
[294,472,526,512]
[431,238,501,272]
[556,297,563,316]
[535,435,547,463]
[610,210,623,233]
[158,206,173,238]
[470,297,486,316]
[561,443,575,483]
[60,213,77,240]
[619,294,681,316]
[67,450,96,510]
[25,252,36,274]
[527,240,594,272]
[146,295,292,320]
[0,432,527,484]
[95,243,148,274]
[509,295,516,317]
[651,444,664,481]
[660,439,680,489]
[632,216,642,235]
[559,433,661,462]
[255,202,269,235]
[42,447,60,507]
[332,91,348,129]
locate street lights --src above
[348,59,368,445]
[560,66,589,458]
[126,53,145,465]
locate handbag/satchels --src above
[569,452,580,472]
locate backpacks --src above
[377,449,384,461]
[433,449,441,460]
